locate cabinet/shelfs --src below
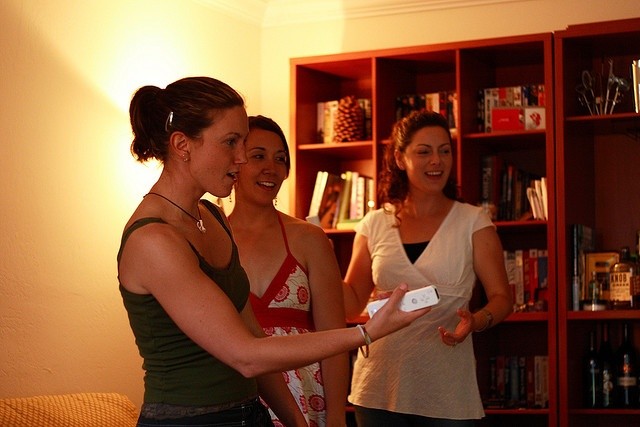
[286,33,558,426]
[556,17,640,426]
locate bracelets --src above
[357,324,370,359]
[471,310,493,333]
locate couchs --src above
[0,392,138,427]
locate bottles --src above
[609,248,636,308]
[580,319,602,408]
[599,321,618,408]
[619,320,640,408]
[588,271,602,301]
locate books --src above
[573,223,596,311]
[308,171,374,224]
[502,250,549,309]
[317,100,373,140]
[480,155,550,222]
[488,351,550,406]
[394,91,458,127]
[478,87,547,130]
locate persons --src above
[116,77,432,427]
[341,108,512,427]
[226,115,350,427]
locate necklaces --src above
[141,193,208,234]
